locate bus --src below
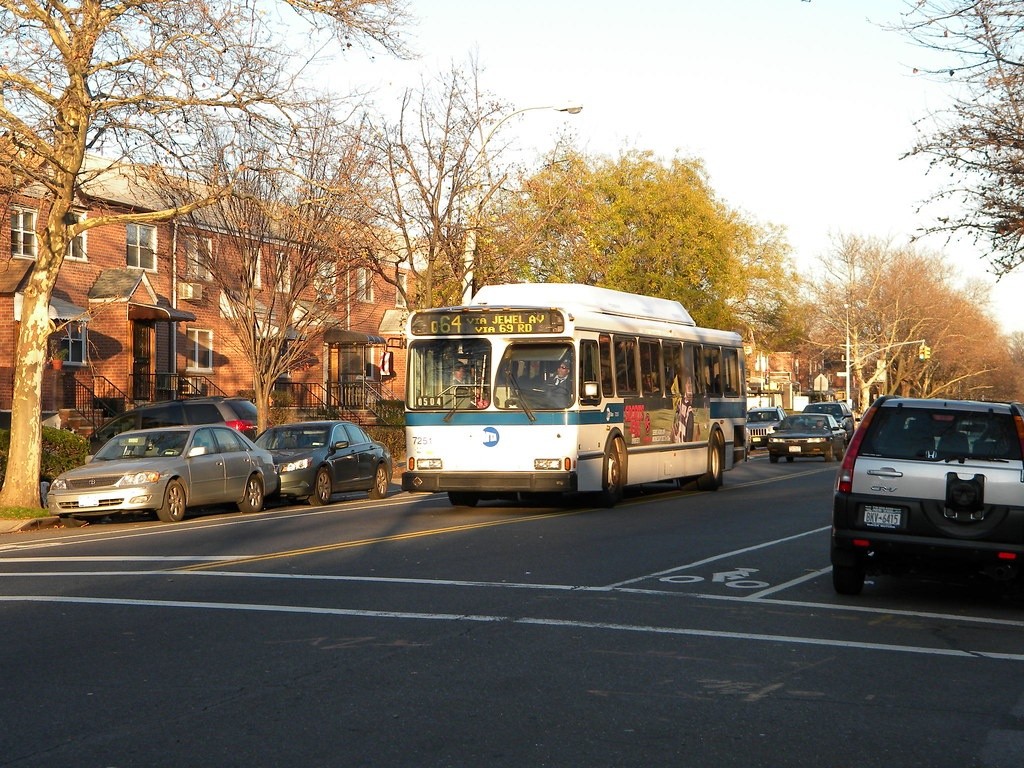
[405,283,746,504]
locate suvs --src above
[802,402,855,444]
[831,396,1024,594]
[86,395,255,441]
[747,406,787,450]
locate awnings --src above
[14,290,90,322]
[127,302,196,322]
[220,290,385,346]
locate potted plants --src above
[48,339,69,370]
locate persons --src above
[812,419,828,430]
[444,357,723,410]
[671,376,694,443]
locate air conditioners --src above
[180,377,202,395]
[179,282,203,300]
[157,372,178,390]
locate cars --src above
[45,424,278,522]
[766,413,846,463]
[251,419,393,506]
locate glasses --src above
[558,365,570,370]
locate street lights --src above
[461,103,583,306]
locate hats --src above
[453,362,468,368]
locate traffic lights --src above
[917,345,931,362]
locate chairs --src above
[890,420,935,456]
[937,433,969,453]
